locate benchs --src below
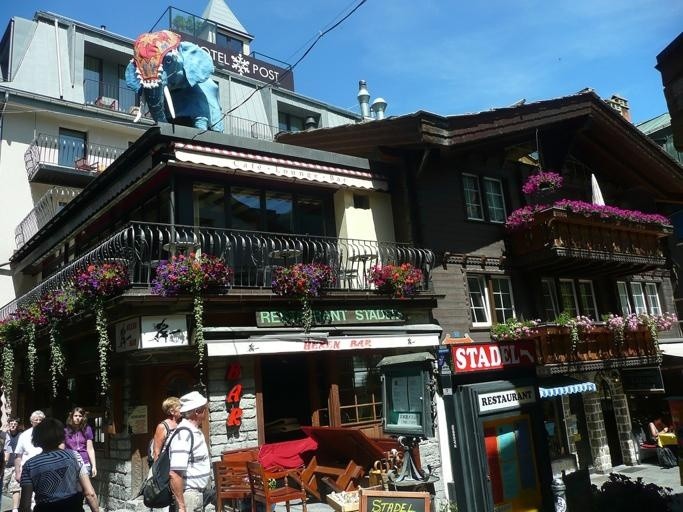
[639,439,657,450]
[220,437,314,480]
[372,435,404,457]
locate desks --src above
[657,432,680,467]
[286,425,388,504]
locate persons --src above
[154,396,182,512]
[0,415,23,512]
[0,430,13,510]
[162,391,211,512]
[14,410,45,510]
[58,407,97,478]
[648,416,680,468]
[18,417,99,512]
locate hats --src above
[176,390,209,413]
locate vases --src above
[536,182,554,196]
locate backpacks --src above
[146,421,170,463]
[138,425,195,508]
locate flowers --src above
[0,265,123,340]
[521,171,560,196]
[489,311,677,349]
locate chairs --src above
[246,460,308,512]
[95,96,115,109]
[212,398,422,510]
[73,158,99,172]
[212,461,260,512]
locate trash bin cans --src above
[550,479,567,512]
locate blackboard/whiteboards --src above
[383,361,424,430]
[361,490,431,512]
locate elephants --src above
[124,29,225,134]
